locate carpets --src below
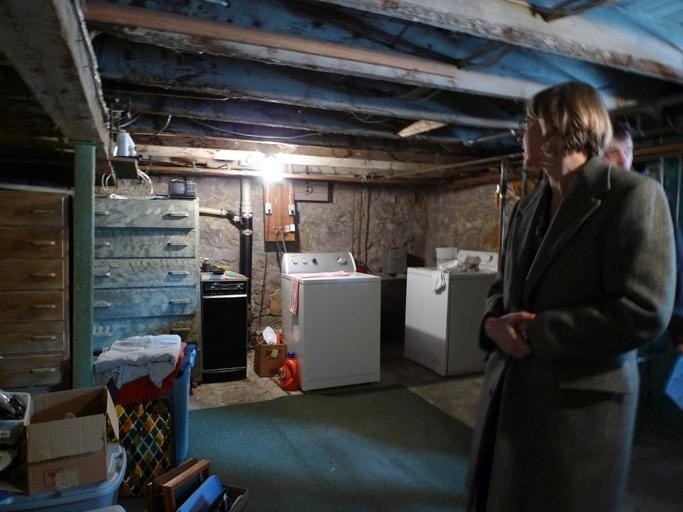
[186,383,483,511]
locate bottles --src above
[201,257,212,272]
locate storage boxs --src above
[0,383,130,510]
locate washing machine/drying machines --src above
[281,252,382,391]
[403,249,498,376]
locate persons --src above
[600,116,636,172]
[467,80,677,509]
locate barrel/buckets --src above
[436,247,458,269]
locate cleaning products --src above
[278,351,298,392]
